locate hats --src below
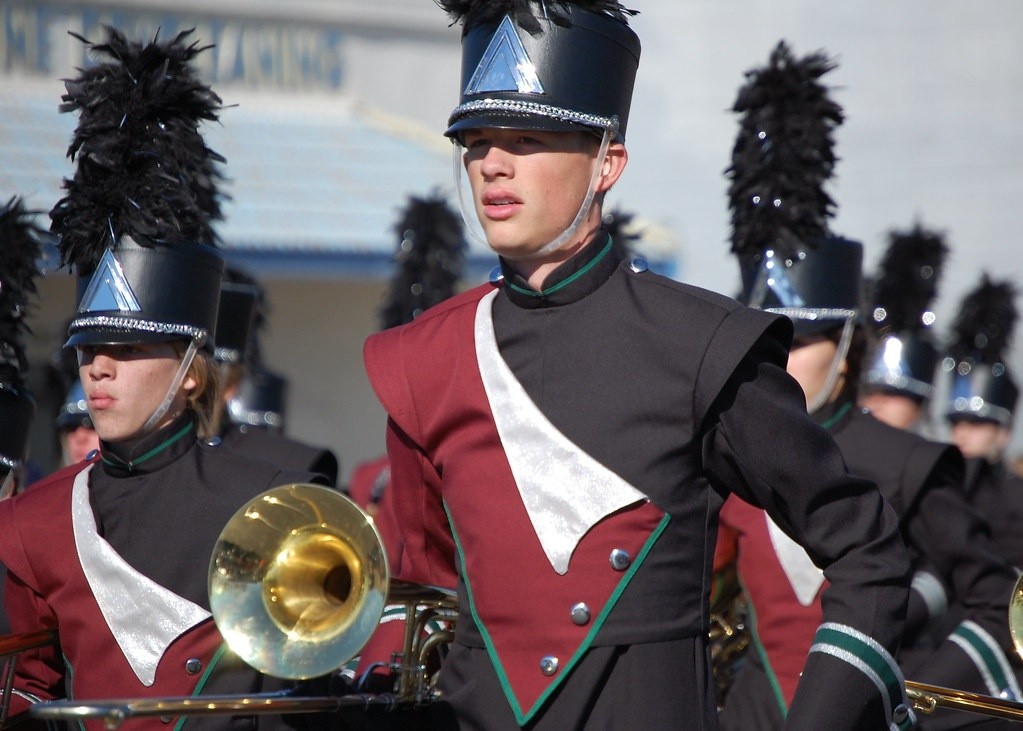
[856,225,950,401]
[48,22,224,355]
[437,0,640,147]
[943,272,1021,428]
[0,195,36,475]
[55,380,94,429]
[720,41,863,335]
[213,258,280,423]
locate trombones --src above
[906,569,1023,731]
[708,584,755,706]
[27,484,463,731]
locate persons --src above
[356,0,913,731]
[0,227,1023,731]
